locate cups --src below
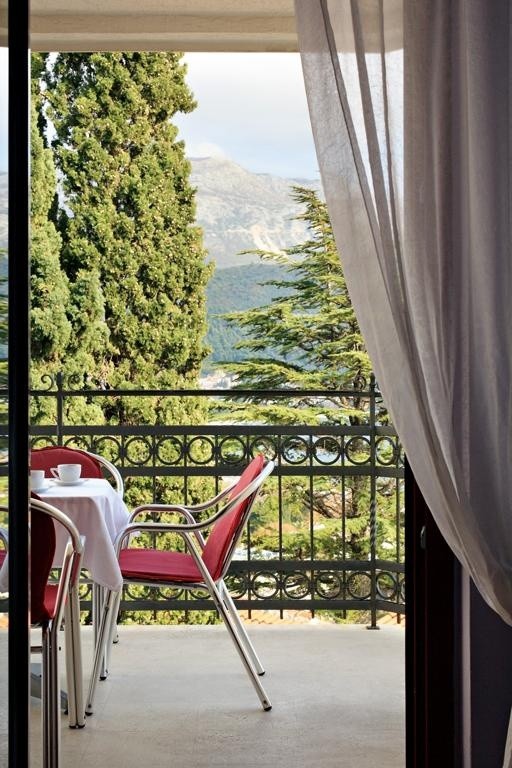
[50,463,81,482]
[29,469,45,487]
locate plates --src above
[52,478,87,486]
[31,484,52,494]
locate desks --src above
[31,473,136,716]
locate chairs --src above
[84,449,283,709]
[27,490,90,729]
[30,440,122,680]
